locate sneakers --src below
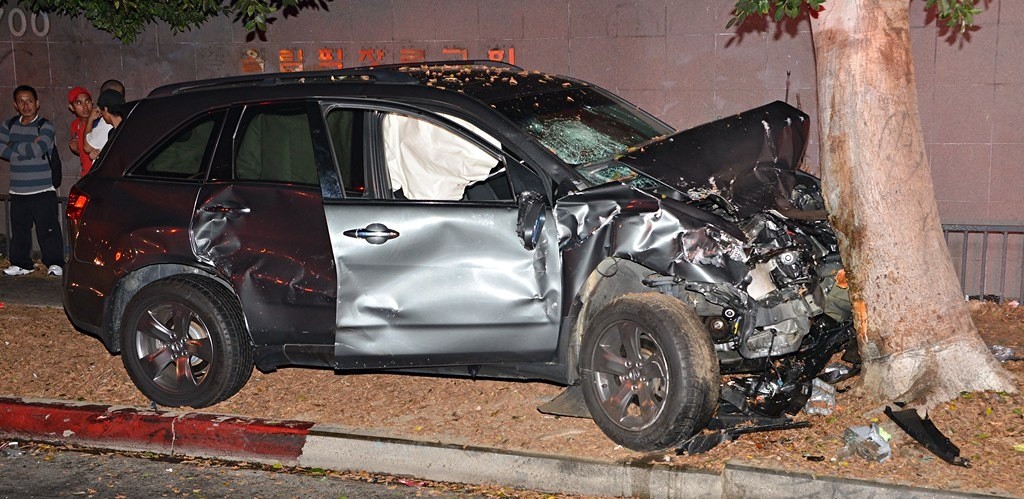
[47,265,63,277]
[3,265,35,275]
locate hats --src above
[67,86,92,103]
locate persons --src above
[1,85,65,278]
[66,85,102,178]
[83,78,126,166]
[90,88,127,162]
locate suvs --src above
[58,59,868,453]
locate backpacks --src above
[8,115,62,188]
